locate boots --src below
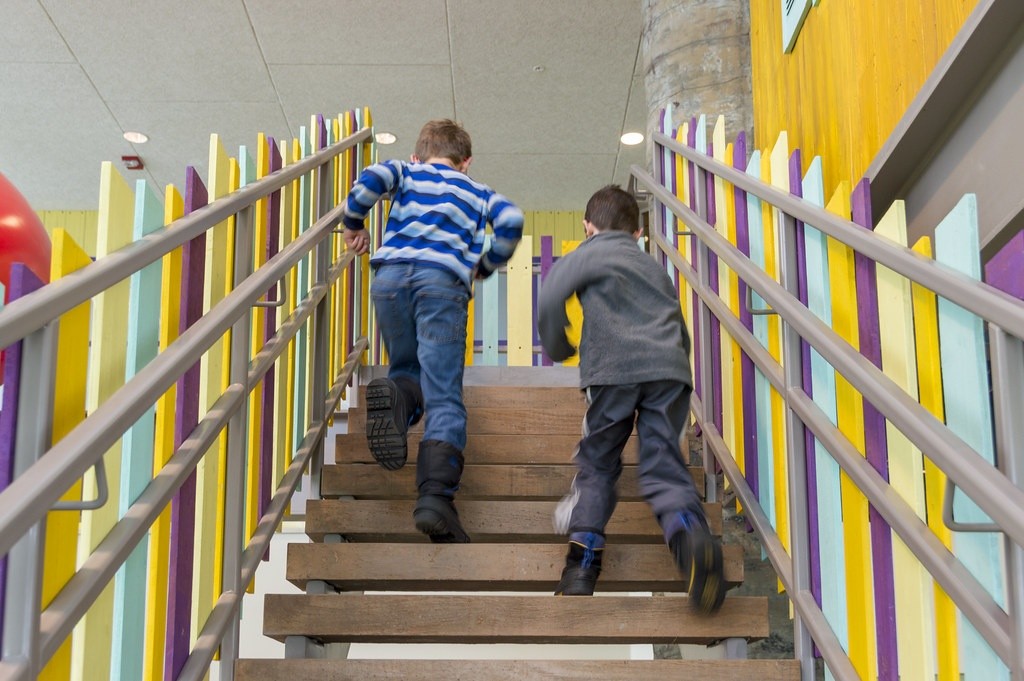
[364,376,425,471]
[675,531,727,615]
[410,438,470,543]
[553,528,607,597]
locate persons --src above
[341,118,525,544]
[537,184,726,614]
